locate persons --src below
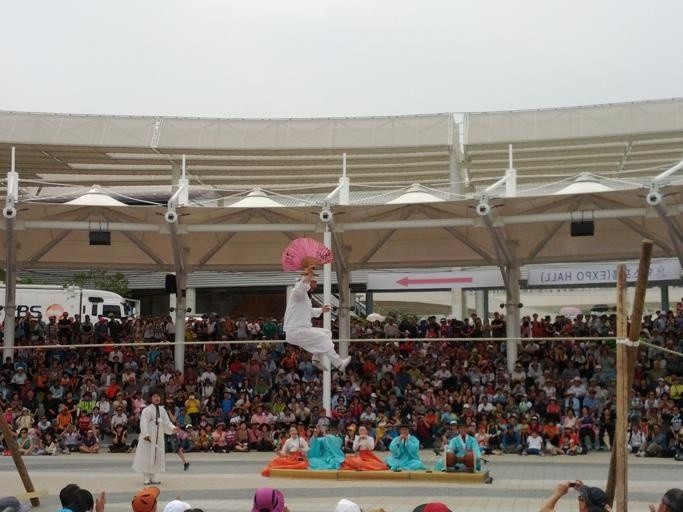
[434,418,484,473]
[385,422,430,471]
[161,494,192,512]
[283,262,352,374]
[250,486,291,512]
[0,495,33,512]
[260,425,309,478]
[333,498,388,512]
[307,415,345,469]
[647,486,683,511]
[411,501,452,512]
[1,302,683,463]
[536,477,615,512]
[71,486,107,512]
[131,386,181,485]
[343,425,391,472]
[56,481,80,512]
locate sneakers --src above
[144,479,161,485]
[183,462,190,472]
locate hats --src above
[14,309,683,432]
[249,488,285,512]
[163,500,190,512]
[579,486,608,512]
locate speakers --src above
[572,221,593,236]
[165,274,176,293]
[89,232,111,245]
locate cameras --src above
[570,483,576,487]
